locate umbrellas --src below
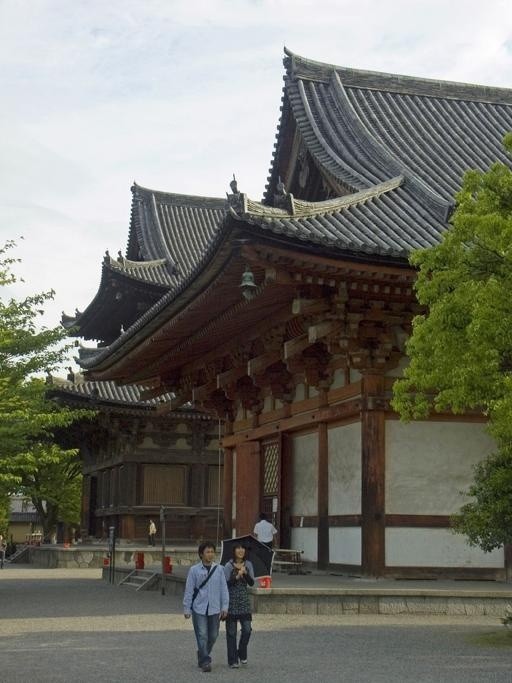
[218,532,275,581]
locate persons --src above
[253,512,278,549]
[223,541,258,671]
[184,540,230,675]
[147,518,157,547]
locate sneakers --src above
[202,663,210,671]
[230,663,239,667]
[240,657,247,663]
